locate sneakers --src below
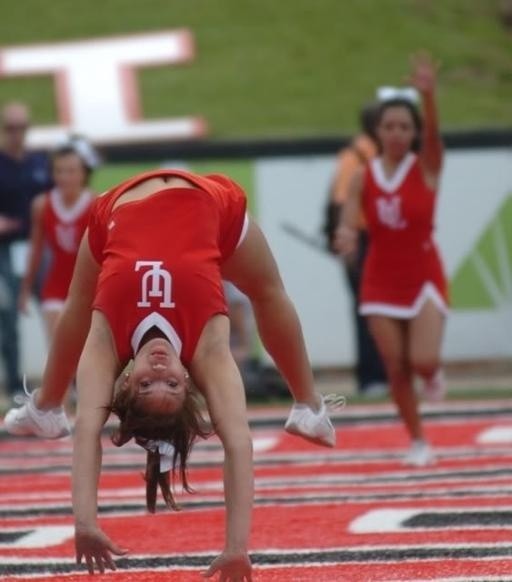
[4,387,71,440]
[426,366,445,399]
[402,441,438,467]
[284,392,336,448]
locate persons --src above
[18,142,99,407]
[1,100,76,398]
[332,44,458,467]
[320,104,391,402]
[4,169,337,581]
